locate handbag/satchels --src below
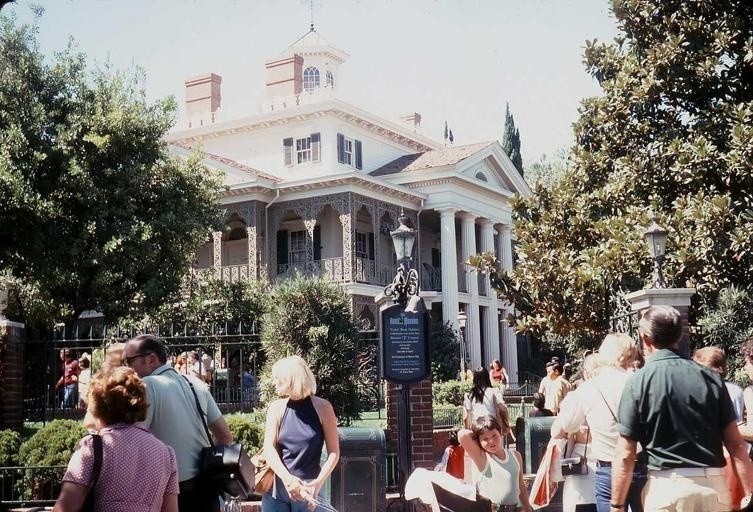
[248,446,275,496]
[199,442,257,501]
[492,394,511,436]
[562,456,588,476]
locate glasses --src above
[121,353,153,366]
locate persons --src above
[261,355,340,512]
[439,360,532,512]
[52,365,180,512]
[165,348,212,383]
[123,333,232,512]
[553,304,753,512]
[56,348,92,411]
[530,355,572,418]
[83,342,126,433]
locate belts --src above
[596,461,612,467]
[646,467,725,479]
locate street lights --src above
[643,210,674,289]
[383,208,419,512]
